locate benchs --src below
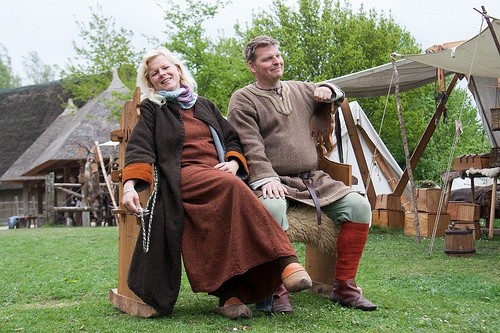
[54,207,92,226]
[109,88,352,318]
[9,216,36,229]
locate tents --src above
[313,6,500,257]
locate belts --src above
[279,172,321,225]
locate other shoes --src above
[281,263,313,292]
[215,297,253,320]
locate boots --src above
[273,293,293,313]
[331,277,377,309]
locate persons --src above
[226,35,379,315]
[120,47,314,320]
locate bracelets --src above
[123,189,135,194]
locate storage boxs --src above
[453,155,490,170]
[372,210,403,228]
[375,195,402,210]
[456,203,480,222]
[403,213,451,238]
[453,222,482,240]
[410,186,446,213]
